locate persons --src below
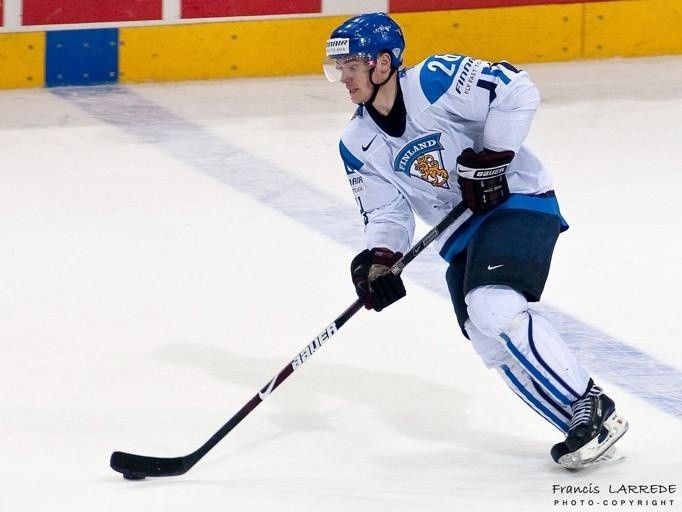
[322,12,616,461]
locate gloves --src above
[350,247,406,312]
[456,146,516,217]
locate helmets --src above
[326,11,406,68]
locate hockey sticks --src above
[111,199,469,475]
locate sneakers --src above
[551,381,616,464]
